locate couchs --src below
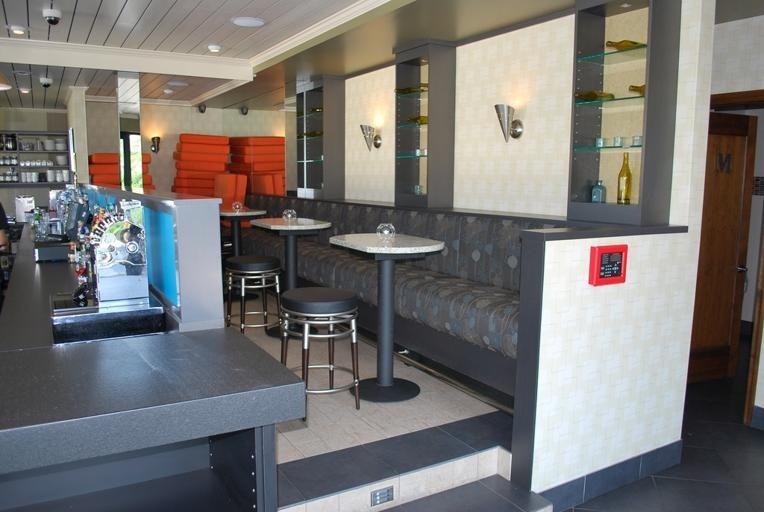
[219,194,568,417]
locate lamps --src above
[495,104,523,144]
[151,136,160,153]
[359,124,382,153]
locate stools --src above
[280,287,360,421]
[223,256,282,334]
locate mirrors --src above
[117,72,143,187]
[222,86,286,197]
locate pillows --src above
[171,133,252,228]
[228,136,285,197]
[88,153,156,195]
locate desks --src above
[328,232,446,403]
[251,217,332,339]
[219,202,269,301]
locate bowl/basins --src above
[21,169,71,183]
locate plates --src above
[43,138,68,151]
[55,154,68,167]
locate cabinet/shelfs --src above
[0,130,73,185]
[394,44,456,208]
[567,0,681,224]
[296,78,345,200]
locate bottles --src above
[407,116,429,124]
[574,90,615,104]
[394,81,428,94]
[591,180,607,204]
[629,83,646,99]
[605,39,647,52]
[0,135,18,183]
[617,151,632,205]
